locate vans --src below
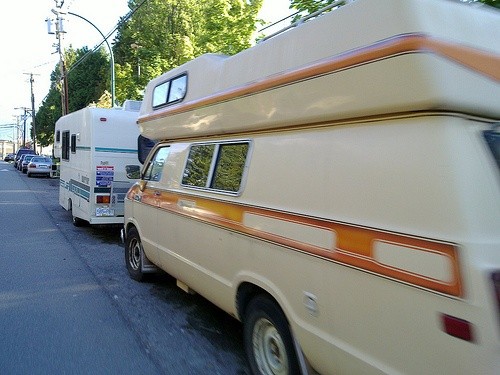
[121,0,499,375]
[15,149,36,169]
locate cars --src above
[4,154,15,162]
[18,154,52,178]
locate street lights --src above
[51,7,117,108]
